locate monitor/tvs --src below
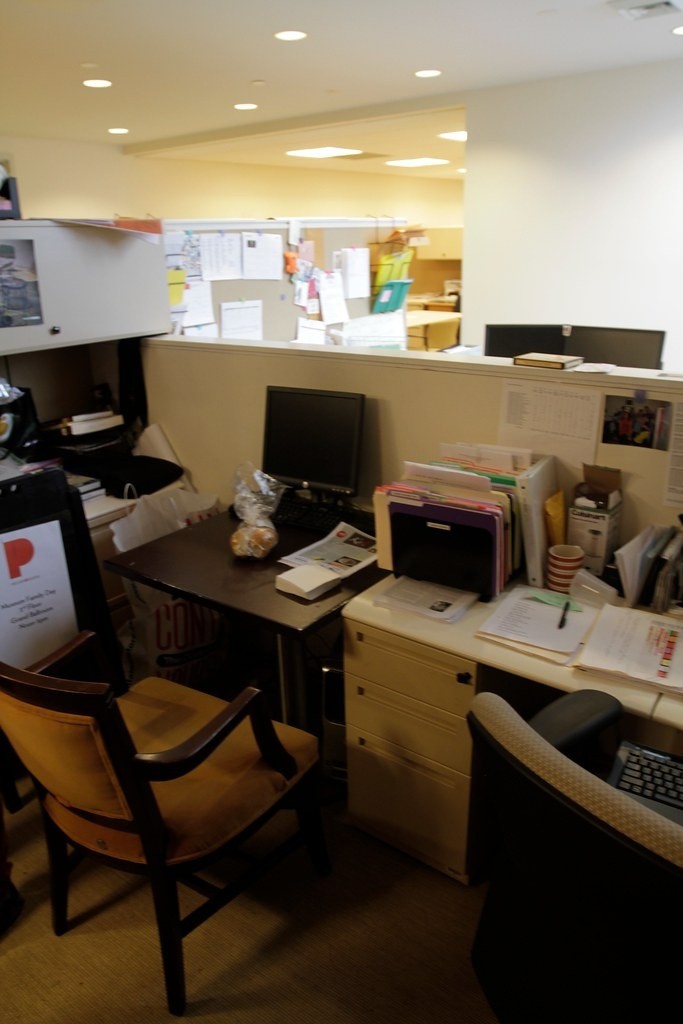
[261,385,365,500]
[485,323,664,370]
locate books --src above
[612,519,683,613]
[22,458,101,493]
[80,488,106,501]
[373,455,564,597]
[46,414,125,436]
[39,408,114,423]
[512,352,584,369]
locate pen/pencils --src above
[558,601,569,629]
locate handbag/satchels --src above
[108,483,223,687]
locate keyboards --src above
[228,495,375,538]
[605,740,683,828]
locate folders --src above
[517,455,558,589]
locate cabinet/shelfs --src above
[343,618,479,891]
[0,217,175,355]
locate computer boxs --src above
[318,658,346,785]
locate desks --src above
[102,507,390,726]
[342,564,682,736]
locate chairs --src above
[461,686,681,1024]
[0,629,328,1015]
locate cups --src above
[546,545,586,594]
[569,569,617,609]
